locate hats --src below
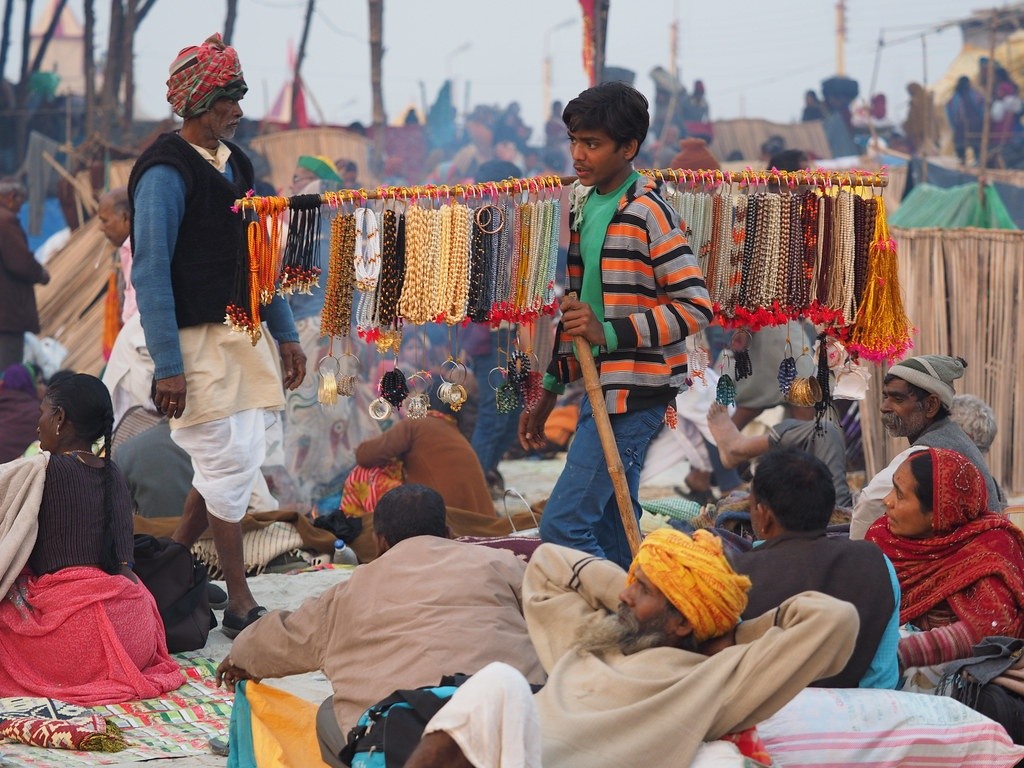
[888,354,969,412]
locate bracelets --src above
[312,349,539,420]
[661,328,873,429]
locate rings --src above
[169,401,177,405]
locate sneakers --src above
[208,583,229,610]
[221,606,267,640]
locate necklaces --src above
[62,450,95,464]
[223,167,918,368]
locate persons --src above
[93,187,155,431]
[518,81,713,572]
[0,57,1024,203]
[403,527,860,768]
[0,370,154,685]
[670,443,901,690]
[0,364,49,467]
[368,159,852,504]
[215,484,548,768]
[0,176,51,374]
[354,380,497,533]
[126,32,306,641]
[849,354,1024,697]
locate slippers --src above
[674,485,716,507]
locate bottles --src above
[333,538,358,566]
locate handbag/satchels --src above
[129,533,217,654]
[336,672,470,768]
